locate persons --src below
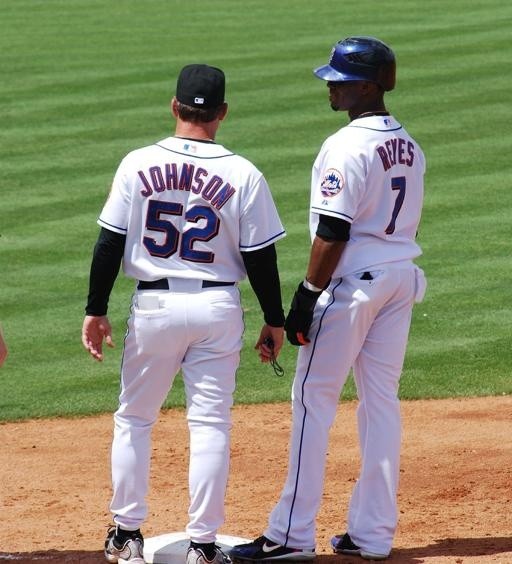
[229,34,428,561]
[80,63,289,564]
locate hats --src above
[176,64,225,109]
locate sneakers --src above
[331,532,388,561]
[104,524,146,564]
[185,544,231,564]
[229,535,317,564]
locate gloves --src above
[284,281,324,346]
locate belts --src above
[137,278,235,290]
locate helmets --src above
[312,37,396,91]
